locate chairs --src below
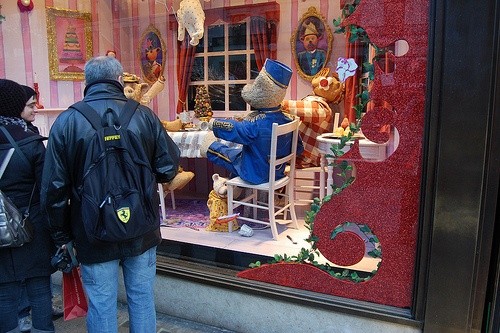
[284,112,341,220]
[226,116,301,240]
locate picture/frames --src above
[45,6,94,81]
[138,24,167,86]
[289,6,334,81]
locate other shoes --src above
[51,305,64,317]
[18,315,32,332]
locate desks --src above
[157,131,222,220]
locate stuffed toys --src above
[206,173,238,231]
[120,71,195,192]
[282,68,347,170]
[210,55,308,194]
[177,0,211,46]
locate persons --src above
[0,79,64,333]
[40,56,180,333]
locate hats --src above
[0,78,36,117]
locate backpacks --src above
[0,146,37,247]
[68,99,160,242]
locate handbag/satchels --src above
[62,246,89,322]
